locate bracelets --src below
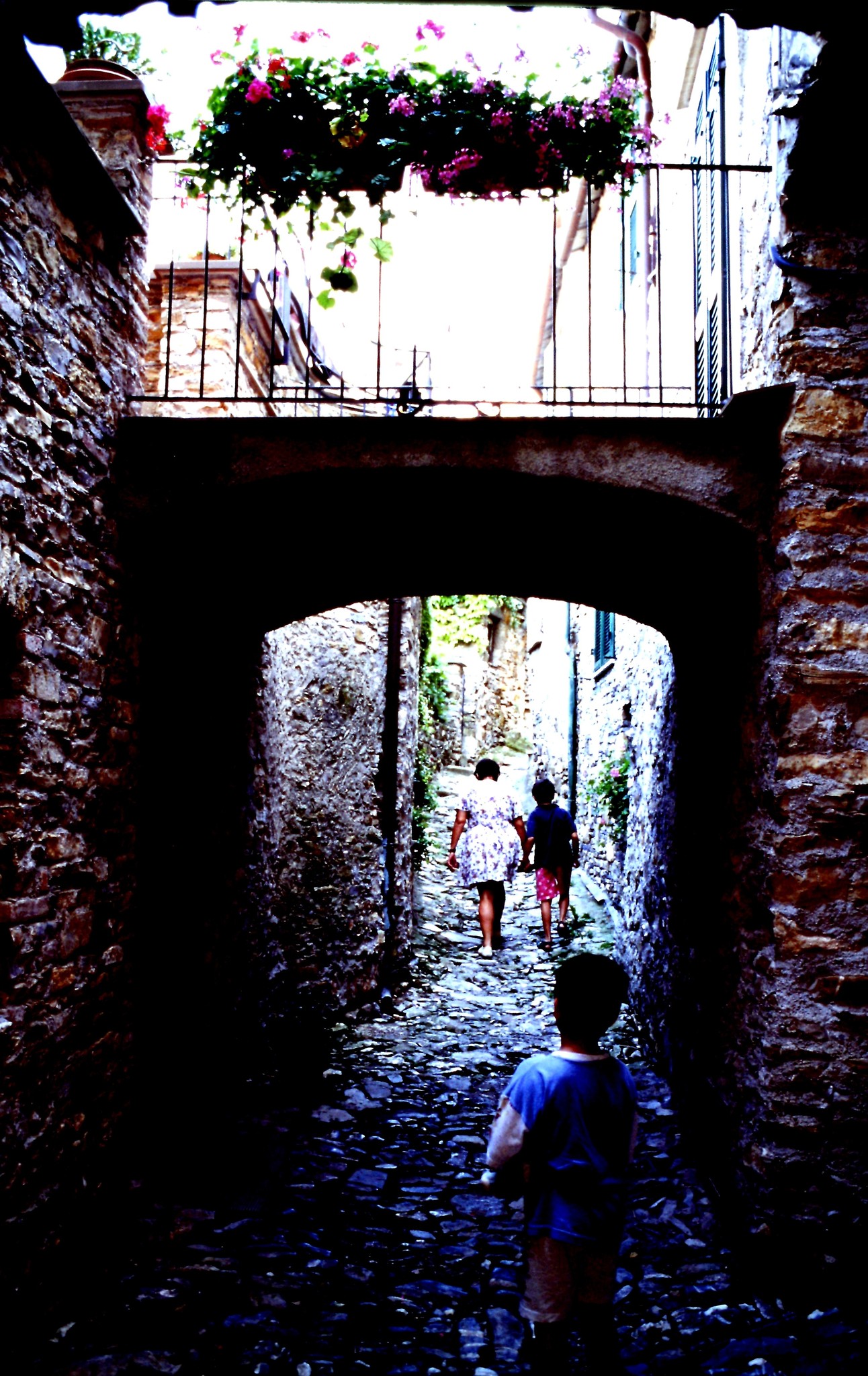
[448,847,456,852]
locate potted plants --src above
[59,23,155,79]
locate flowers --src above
[130,20,670,301]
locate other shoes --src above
[477,944,493,957]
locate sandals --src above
[557,921,571,940]
[536,938,553,951]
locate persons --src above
[447,758,530,957]
[482,952,639,1376]
[517,779,580,952]
